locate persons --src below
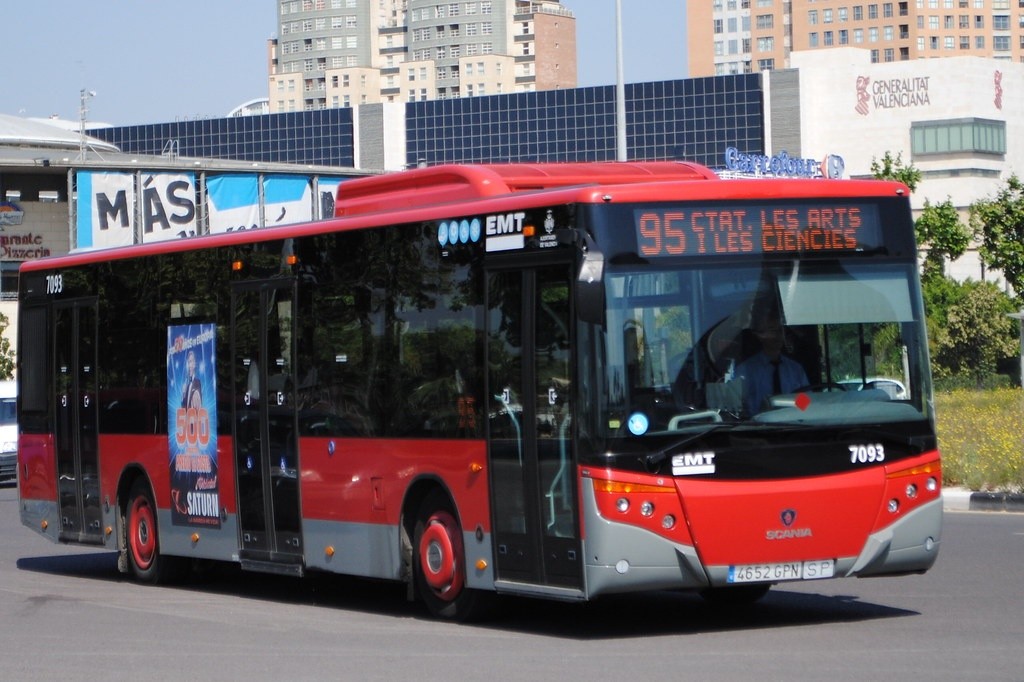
[727,320,811,416]
[180,350,202,434]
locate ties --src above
[772,366,782,396]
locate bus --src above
[17,160,943,620]
